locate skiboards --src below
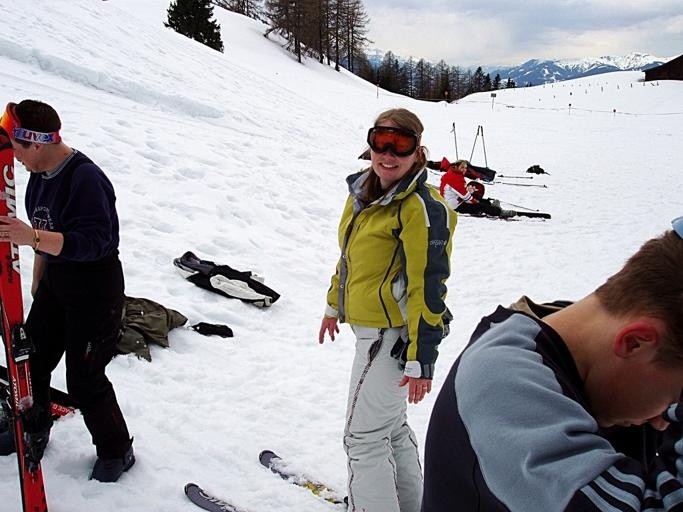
[183,451,346,512]
[426,172,555,220]
[2,113,49,510]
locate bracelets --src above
[34,229,40,251]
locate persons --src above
[1,99,135,483]
[319,107,456,512]
[420,216,682,510]
[441,160,517,218]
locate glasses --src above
[368,127,418,157]
[0,102,62,145]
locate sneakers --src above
[1,429,49,455]
[89,437,135,482]
[500,209,516,219]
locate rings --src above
[5,231,9,238]
